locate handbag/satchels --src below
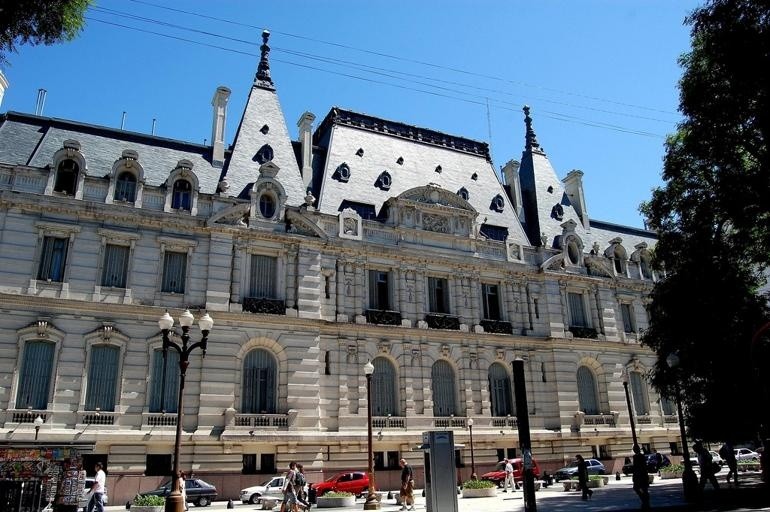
[294,472,306,486]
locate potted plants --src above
[462,480,498,498]
[591,475,604,488]
[659,464,685,479]
[130,493,166,512]
[316,490,356,508]
[735,459,760,472]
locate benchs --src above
[558,478,591,492]
[516,480,546,492]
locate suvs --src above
[481,457,540,492]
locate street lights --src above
[619,369,638,446]
[361,357,382,510]
[156,309,215,512]
[466,416,478,484]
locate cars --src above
[137,477,218,509]
[310,472,369,500]
[238,475,310,505]
[622,447,762,477]
[557,456,608,488]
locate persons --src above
[654,449,664,470]
[399,458,416,510]
[279,460,312,511]
[759,439,770,483]
[631,444,651,509]
[691,442,724,499]
[82,461,107,511]
[720,441,739,484]
[501,459,516,492]
[625,456,631,477]
[571,453,594,501]
[178,469,190,510]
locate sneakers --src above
[581,492,593,500]
[399,506,415,510]
[303,503,312,512]
